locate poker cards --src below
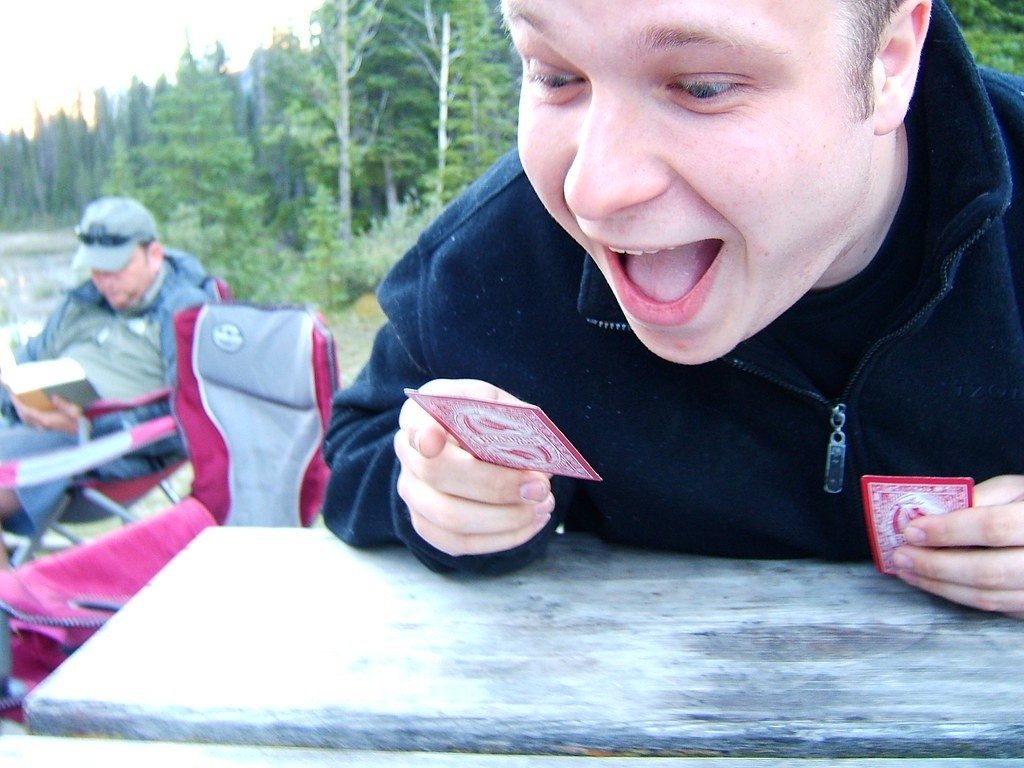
[859,474,975,577]
[402,388,604,483]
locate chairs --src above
[0,302,344,740]
[1,274,233,562]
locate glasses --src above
[74,225,137,247]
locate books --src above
[0,342,101,414]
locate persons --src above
[319,0,1024,619]
[0,195,222,570]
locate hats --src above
[69,196,160,272]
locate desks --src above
[22,525,1024,763]
